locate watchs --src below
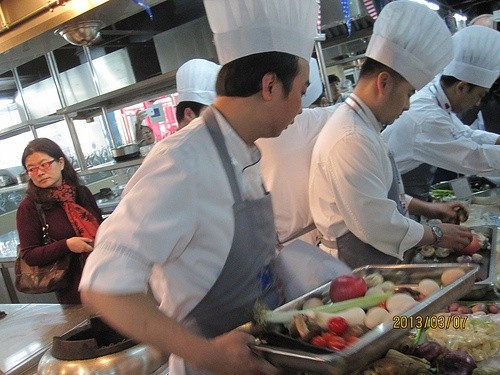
[429,224,444,246]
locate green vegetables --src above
[430,189,488,197]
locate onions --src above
[412,341,478,375]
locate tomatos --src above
[310,316,359,351]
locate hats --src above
[203,0,318,66]
[173,58,223,107]
[364,0,455,91]
[301,57,323,107]
[442,25,500,89]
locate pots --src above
[110,139,146,162]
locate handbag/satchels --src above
[15,189,71,294]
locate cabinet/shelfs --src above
[1,0,334,303]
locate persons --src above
[77,23,311,375]
[379,52,500,221]
[254,57,352,246]
[174,57,224,132]
[308,36,473,271]
[456,14,500,134]
[133,109,156,147]
[16,138,107,306]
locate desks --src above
[2,303,94,375]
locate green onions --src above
[252,289,411,323]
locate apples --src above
[329,275,367,303]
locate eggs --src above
[386,294,418,314]
[441,269,465,286]
[364,306,389,328]
[419,279,440,296]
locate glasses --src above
[143,131,152,136]
[26,160,57,175]
